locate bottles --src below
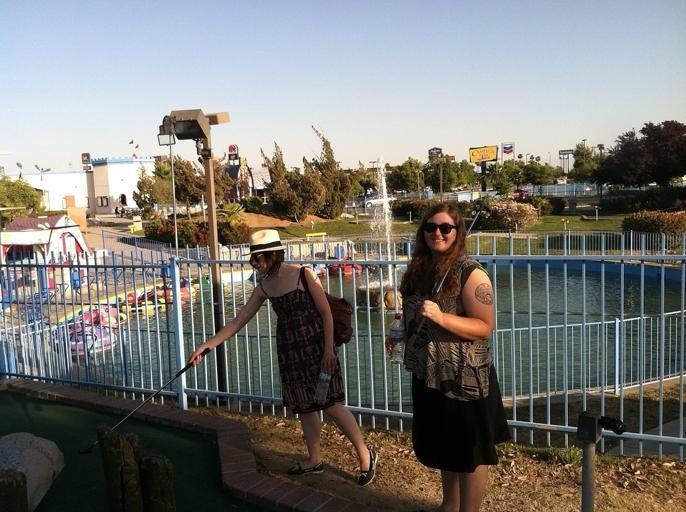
[388,313,406,364]
[313,371,331,405]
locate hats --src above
[241,229,286,257]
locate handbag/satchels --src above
[325,292,353,345]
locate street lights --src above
[583,138,605,205]
[158,109,230,406]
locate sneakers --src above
[288,460,323,476]
[359,450,378,486]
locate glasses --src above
[424,223,459,233]
[253,254,266,261]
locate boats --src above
[302,261,362,277]
[48,277,210,356]
[304,257,363,277]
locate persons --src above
[384,204,511,512]
[114,205,126,218]
[187,229,379,487]
